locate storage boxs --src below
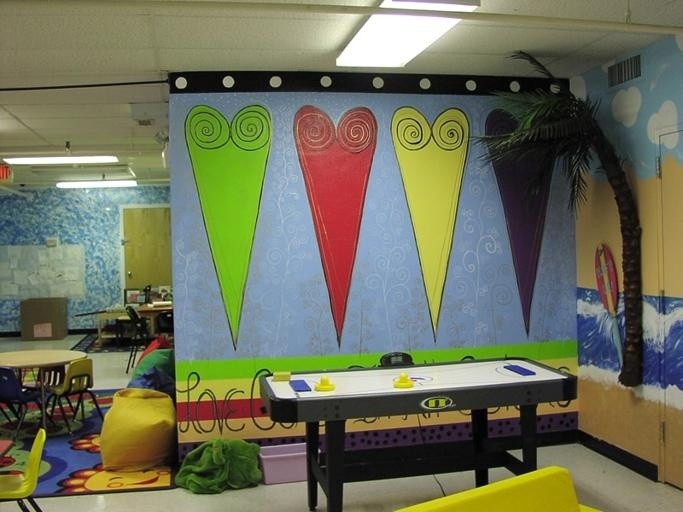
[20,297,68,341]
[259,442,321,485]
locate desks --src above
[0,350,87,430]
[260,355,578,511]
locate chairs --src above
[0,428,46,512]
[0,359,103,440]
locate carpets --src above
[0,389,177,499]
